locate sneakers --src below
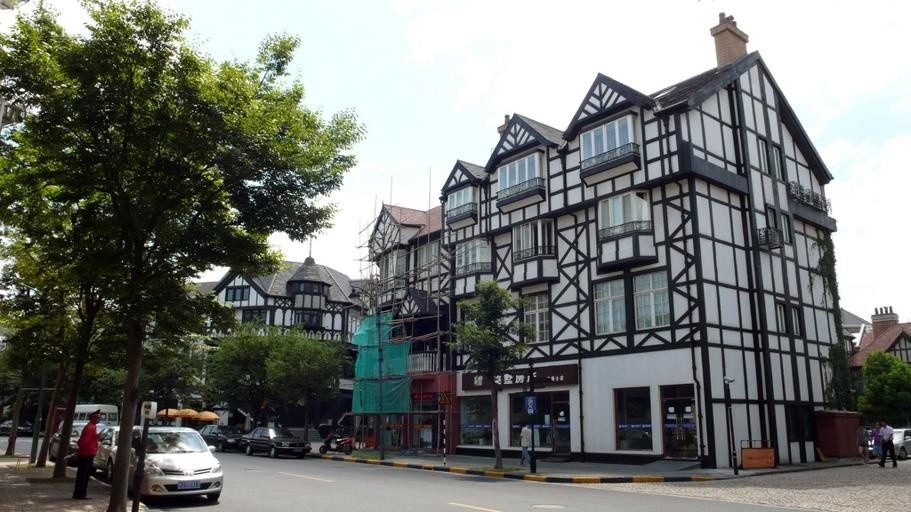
[72,496,93,500]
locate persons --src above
[518,424,531,466]
[877,420,897,468]
[72,409,102,500]
[856,423,871,463]
[870,420,882,458]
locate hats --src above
[88,409,101,418]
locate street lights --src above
[346,280,391,460]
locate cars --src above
[49,404,314,501]
[1,419,33,437]
[865,428,911,462]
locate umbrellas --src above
[192,411,220,424]
[173,409,197,426]
[158,408,178,418]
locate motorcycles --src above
[319,430,353,456]
